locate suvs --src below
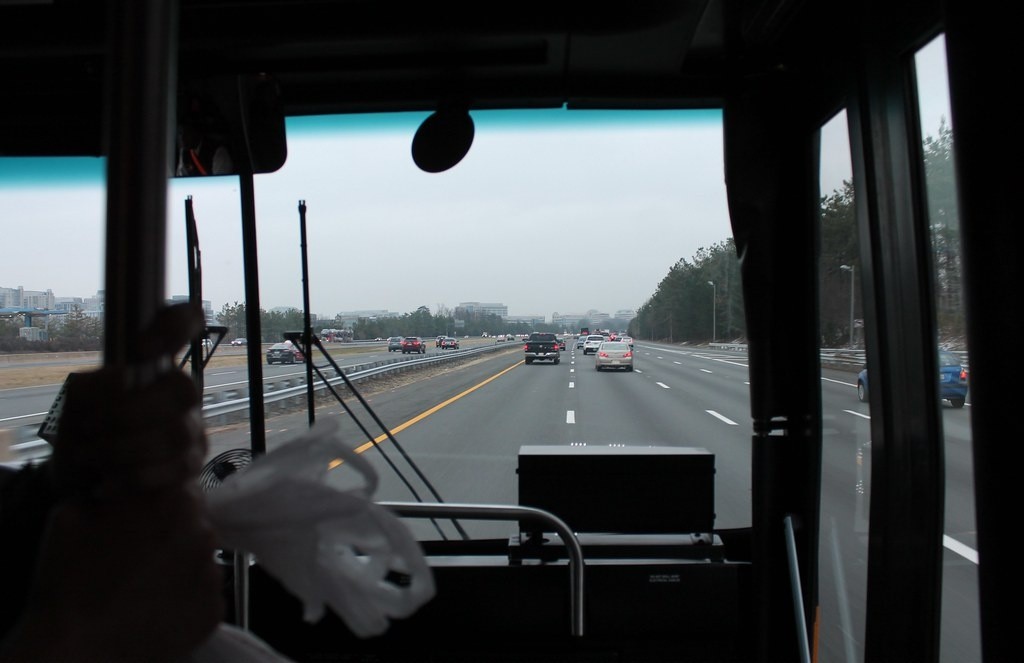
[231,337,247,347]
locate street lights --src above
[707,281,717,343]
[838,264,855,346]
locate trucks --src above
[319,328,354,342]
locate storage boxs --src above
[517,446,716,533]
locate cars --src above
[386,336,405,352]
[202,338,213,347]
[441,337,460,350]
[557,337,566,351]
[497,336,506,342]
[435,335,448,347]
[619,337,634,348]
[857,347,969,408]
[401,336,427,354]
[507,335,514,341]
[592,341,634,372]
[573,335,579,340]
[285,339,306,363]
[602,331,628,341]
[522,336,529,342]
[576,335,588,350]
[266,343,297,365]
[583,335,605,354]
[375,337,383,341]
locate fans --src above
[197,449,261,492]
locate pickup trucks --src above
[522,332,560,365]
